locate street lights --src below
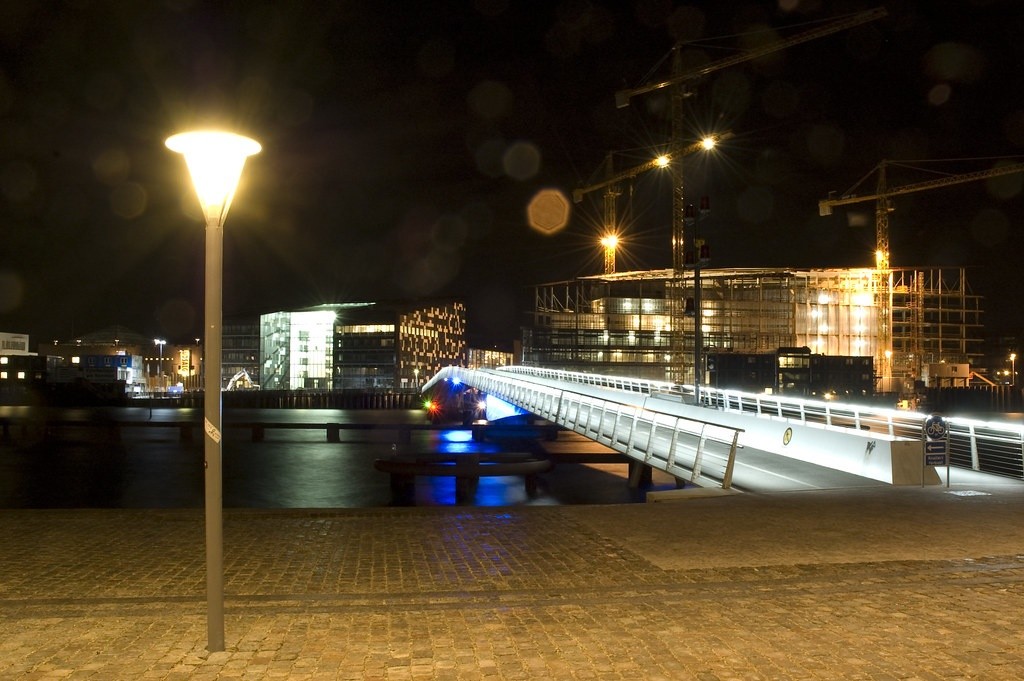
[684,195,714,399]
[165,128,265,655]
[154,338,167,399]
[1009,352,1017,387]
[413,368,420,387]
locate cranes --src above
[572,126,738,275]
[819,154,1024,392]
[614,5,893,384]
